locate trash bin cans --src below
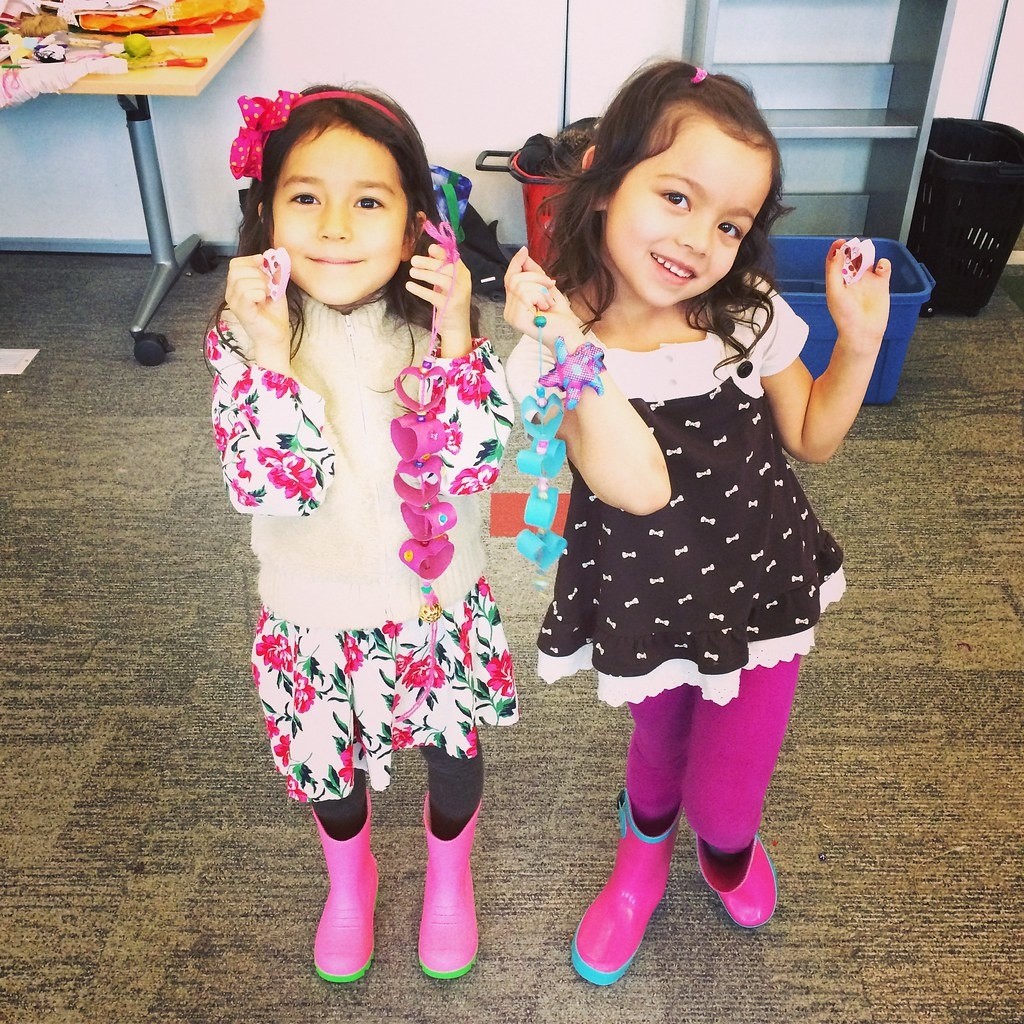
[905,117,1024,317]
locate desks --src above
[0,0,266,365]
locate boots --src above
[314,787,378,982]
[570,788,682,985]
[696,833,777,929]
[418,791,483,979]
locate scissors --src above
[128,56,207,68]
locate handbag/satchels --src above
[429,165,472,244]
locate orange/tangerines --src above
[124,33,151,58]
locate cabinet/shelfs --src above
[683,0,961,248]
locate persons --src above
[206,84,521,982]
[502,57,890,988]
